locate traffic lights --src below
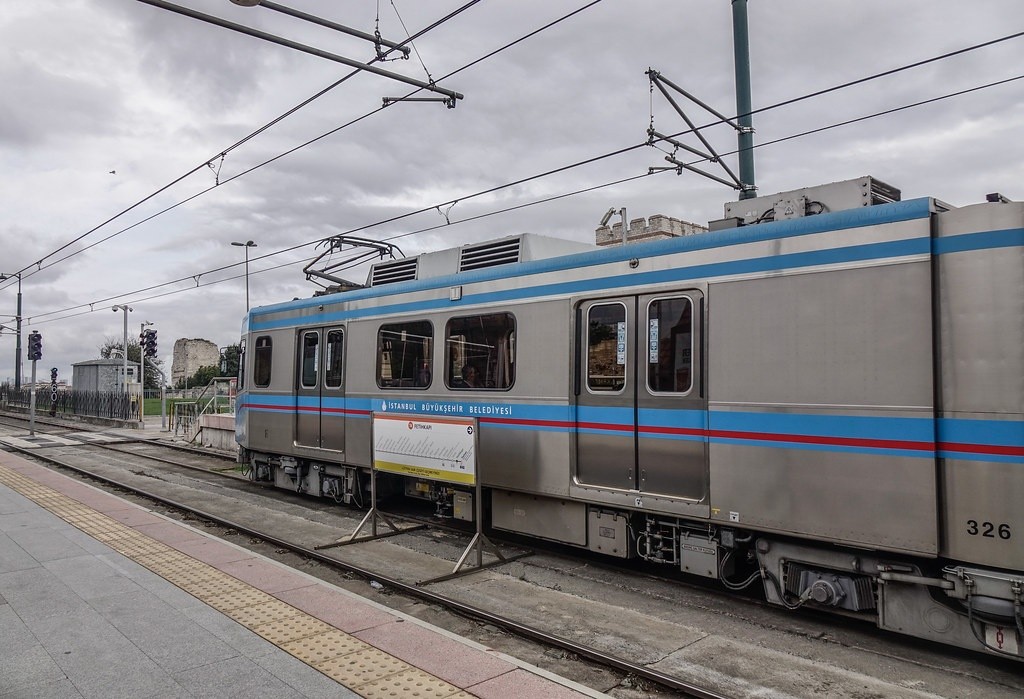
[51,367,58,380]
[146,329,157,357]
[28,334,43,360]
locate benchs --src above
[390,378,419,387]
[450,379,485,388]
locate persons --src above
[457,366,484,390]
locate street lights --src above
[113,305,132,392]
[231,240,258,313]
[0,271,21,396]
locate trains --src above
[234,192,1024,664]
